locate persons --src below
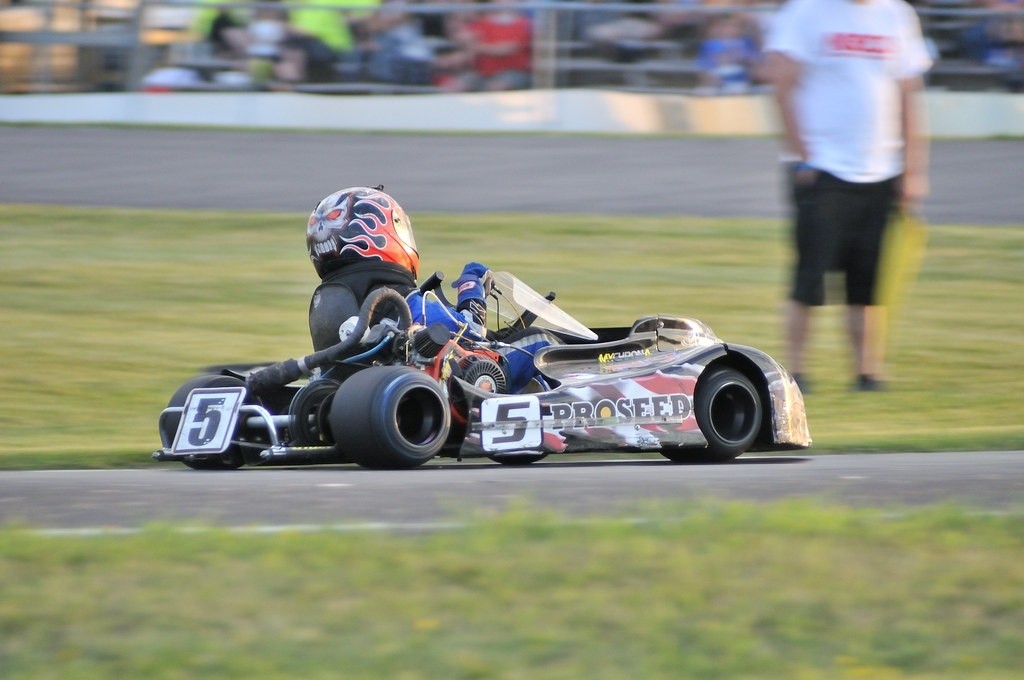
[914,1,1024,94]
[307,185,587,395]
[763,0,932,391]
[143,0,772,94]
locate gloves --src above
[451,261,495,311]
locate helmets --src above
[306,184,419,281]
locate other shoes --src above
[858,374,890,392]
[790,373,810,396]
